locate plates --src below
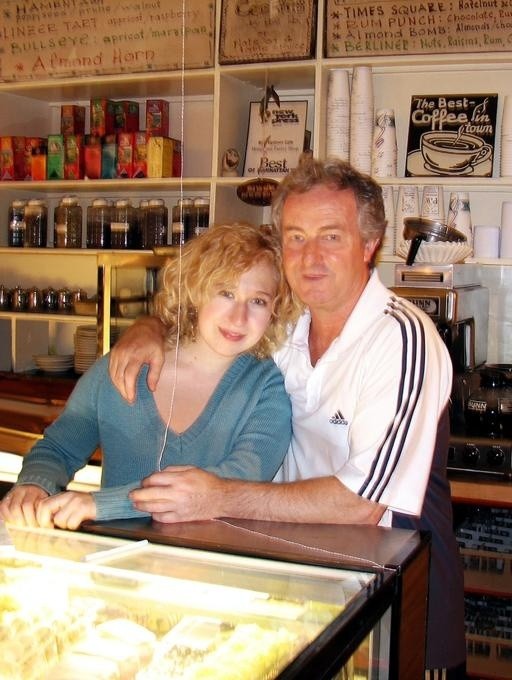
[73,326,97,376]
[32,354,74,374]
[393,240,472,265]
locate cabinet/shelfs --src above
[0,1,510,542]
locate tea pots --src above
[0,284,87,313]
[465,366,512,437]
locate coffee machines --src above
[384,286,490,433]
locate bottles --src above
[8,199,208,248]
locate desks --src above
[0,477,422,680]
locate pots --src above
[401,216,467,266]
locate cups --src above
[75,300,96,315]
[326,65,397,180]
[499,94,512,177]
[119,298,144,318]
[377,184,472,256]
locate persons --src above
[109,153,467,680]
[0,222,306,530]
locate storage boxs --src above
[2,4,214,83]
[218,1,315,64]
[326,2,510,58]
